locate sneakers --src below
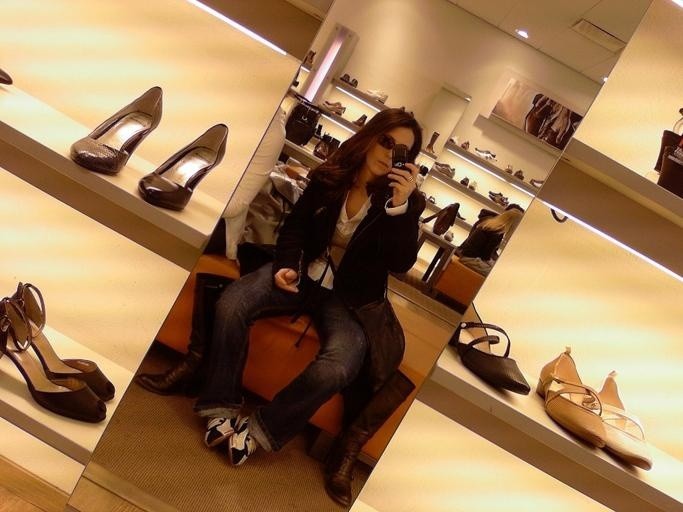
[203,409,262,466]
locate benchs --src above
[155,253,347,437]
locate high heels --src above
[0,282,114,422]
[71,87,162,174]
[139,124,228,211]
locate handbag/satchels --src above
[423,203,459,235]
[286,94,330,145]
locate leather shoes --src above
[535,348,654,470]
[449,302,530,396]
[415,132,545,207]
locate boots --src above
[134,273,236,394]
[314,135,340,160]
[323,75,388,125]
[325,371,416,507]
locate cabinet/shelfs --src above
[415,138,683,512]
[0,0,302,512]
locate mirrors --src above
[66,0,650,512]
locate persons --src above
[204,106,287,261]
[452,207,524,264]
[191,108,426,467]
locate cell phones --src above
[392,144,407,170]
